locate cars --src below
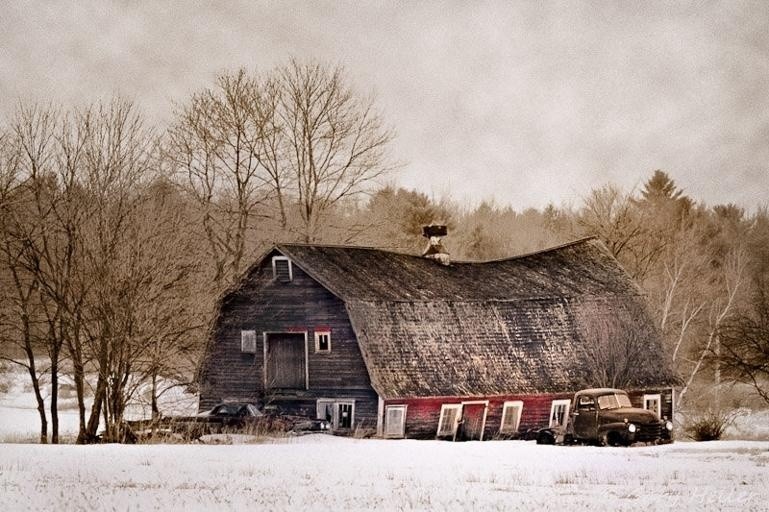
[170,401,331,440]
